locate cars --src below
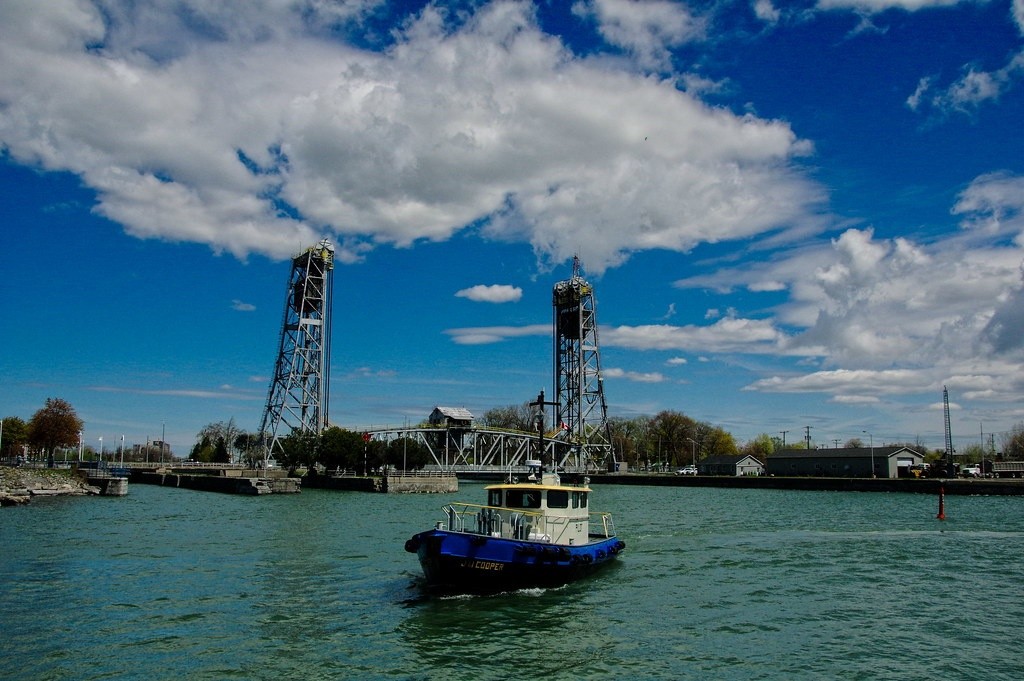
[642,462,698,475]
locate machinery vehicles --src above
[910,385,959,477]
[963,458,1024,479]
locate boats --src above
[405,460,627,595]
